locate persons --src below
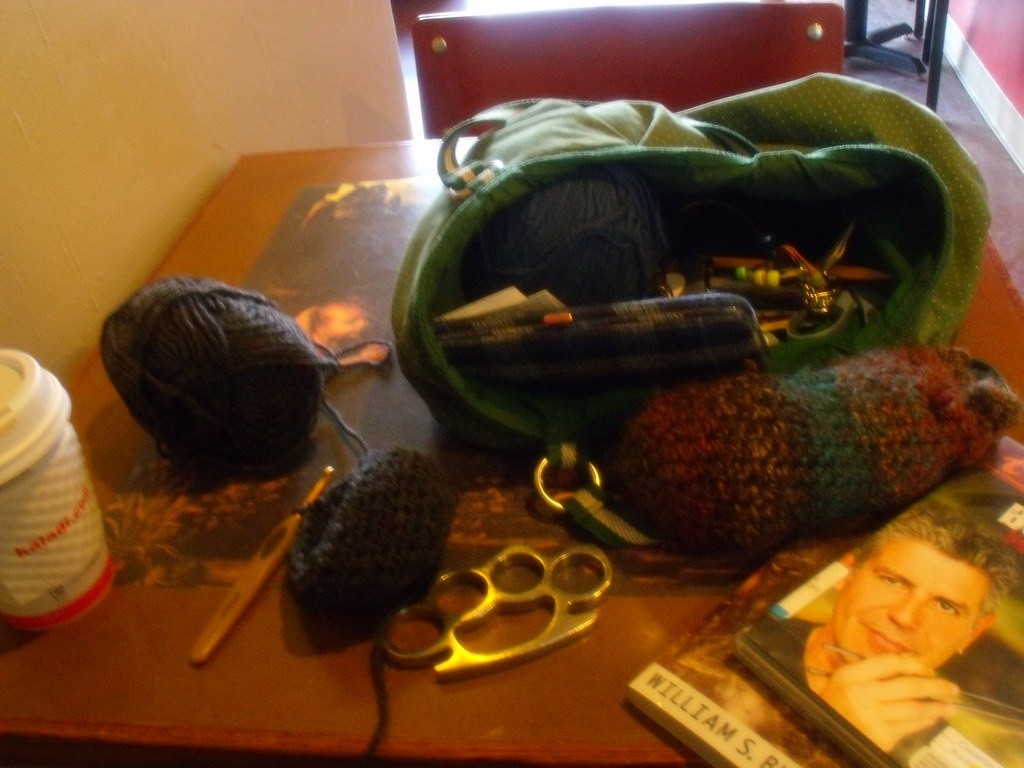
[751,499,1020,750]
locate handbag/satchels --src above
[392,74,994,546]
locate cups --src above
[0,342,115,632]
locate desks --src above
[1,136,1023,768]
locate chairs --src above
[412,4,845,140]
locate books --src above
[624,436,1024,768]
